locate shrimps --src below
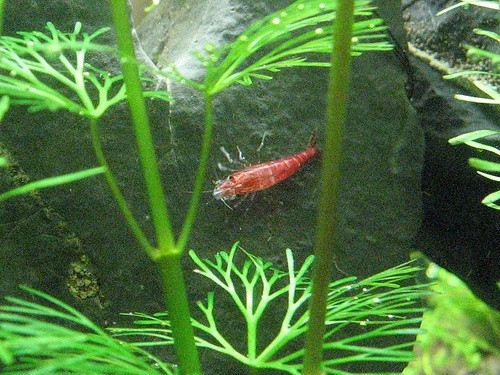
[184,126,324,213]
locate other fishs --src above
[0,142,112,311]
